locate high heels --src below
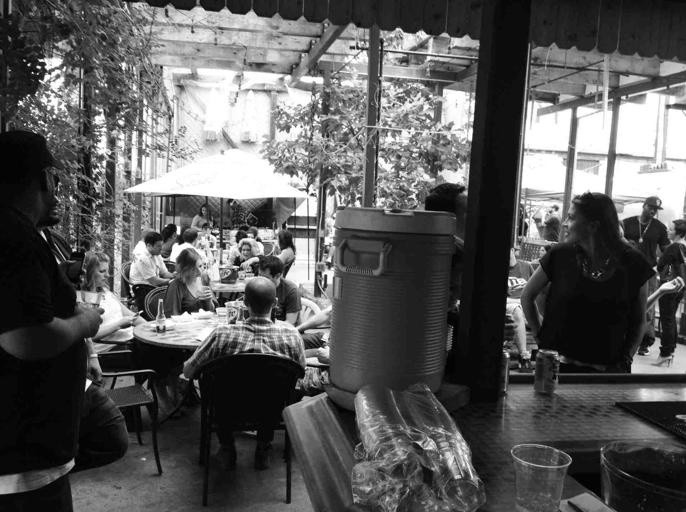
[651,352,674,367]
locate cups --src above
[510,444,573,510]
[76,289,100,311]
[354,385,484,512]
[216,307,228,324]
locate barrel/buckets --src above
[218,265,239,284]
[330,205,457,396]
[600,440,686,512]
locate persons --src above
[502,192,686,374]
[36,166,467,471]
[1,129,105,511]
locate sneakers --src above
[214,448,236,470]
[639,345,650,355]
[518,354,533,373]
[254,445,271,469]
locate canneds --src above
[498,349,511,393]
[534,349,560,393]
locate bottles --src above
[155,299,167,333]
[235,306,245,325]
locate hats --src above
[0,130,63,181]
[645,196,663,209]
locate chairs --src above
[119,242,321,319]
[90,352,161,477]
[301,327,328,396]
[194,352,304,507]
[90,338,152,391]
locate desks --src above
[133,311,233,425]
